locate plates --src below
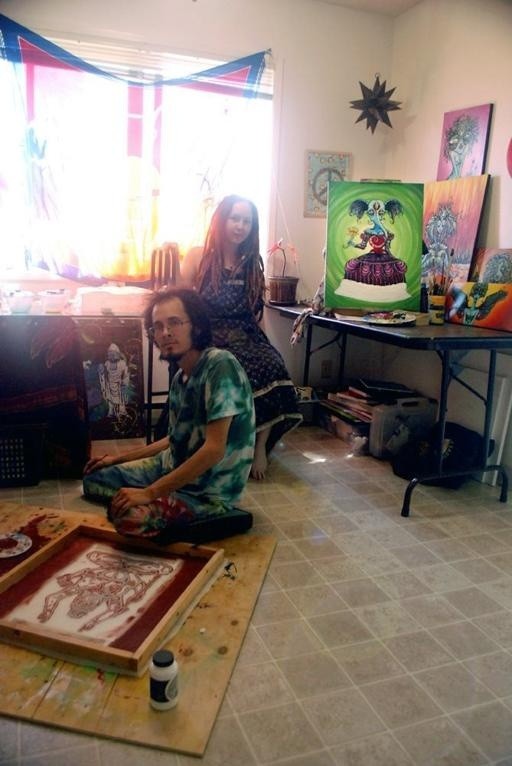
[0,531,32,559]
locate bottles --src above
[151,650,180,711]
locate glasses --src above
[150,320,191,336]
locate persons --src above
[81,285,259,538]
[177,194,304,481]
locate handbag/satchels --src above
[369,397,439,458]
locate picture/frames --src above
[302,148,354,219]
[268,275,301,308]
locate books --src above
[327,377,413,420]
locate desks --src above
[0,310,146,493]
[267,299,511,513]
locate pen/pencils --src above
[89,455,107,467]
[429,248,455,295]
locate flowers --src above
[265,239,304,280]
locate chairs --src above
[147,241,181,439]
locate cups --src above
[4,288,71,317]
[427,295,446,326]
